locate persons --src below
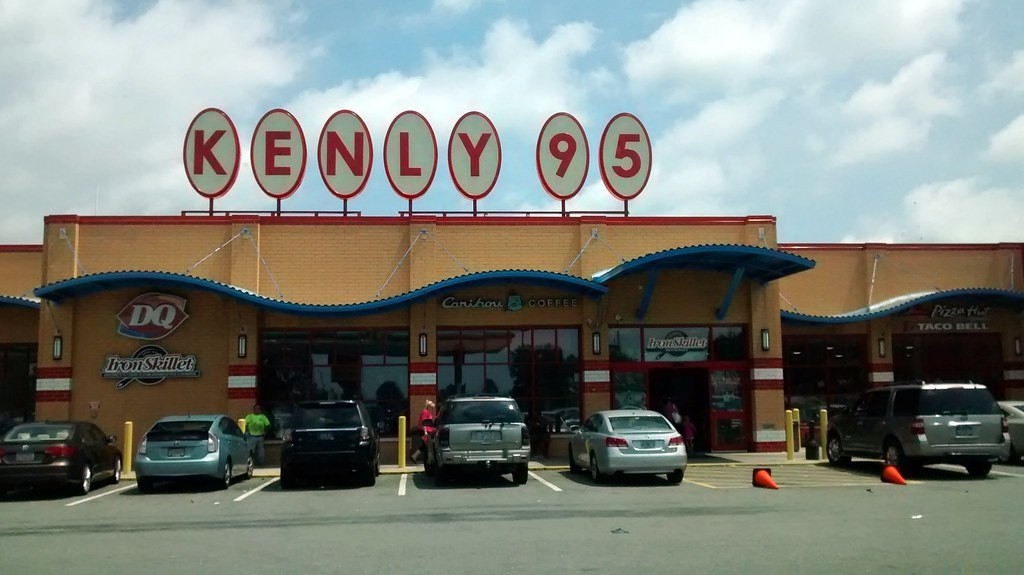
[682,416,697,458]
[245,403,271,468]
[410,399,437,464]
[16,366,37,422]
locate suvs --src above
[280,400,380,490]
[427,393,531,485]
[366,403,392,438]
[826,380,1023,476]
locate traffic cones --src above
[752,468,779,490]
[881,465,907,485]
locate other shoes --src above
[410,457,416,464]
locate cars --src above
[522,407,580,433]
[712,395,742,431]
[134,415,254,494]
[0,421,123,494]
[568,410,688,484]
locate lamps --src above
[878,338,887,357]
[238,334,247,357]
[419,333,427,356]
[592,333,601,355]
[761,329,770,351]
[1013,337,1023,356]
[53,336,62,360]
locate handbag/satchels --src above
[672,404,682,423]
